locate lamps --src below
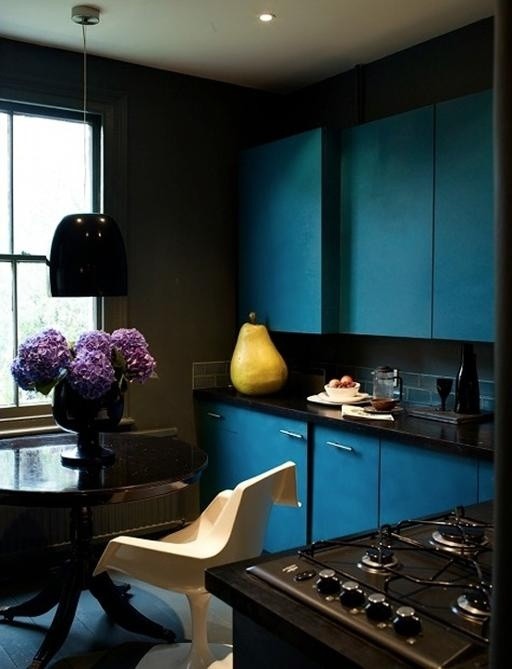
[50,6,130,298]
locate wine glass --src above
[436,378,454,414]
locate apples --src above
[341,376,352,383]
[329,379,339,388]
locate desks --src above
[0,434,209,669]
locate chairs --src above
[93,463,302,669]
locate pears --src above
[231,312,288,396]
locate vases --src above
[52,382,125,468]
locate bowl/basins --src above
[371,398,400,411]
[323,382,361,400]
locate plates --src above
[363,406,404,414]
[306,391,373,405]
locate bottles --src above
[453,343,481,415]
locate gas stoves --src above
[245,503,494,669]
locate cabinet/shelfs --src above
[198,402,495,556]
[241,88,496,346]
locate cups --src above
[370,366,403,402]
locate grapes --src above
[334,381,356,388]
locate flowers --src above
[11,327,157,399]
